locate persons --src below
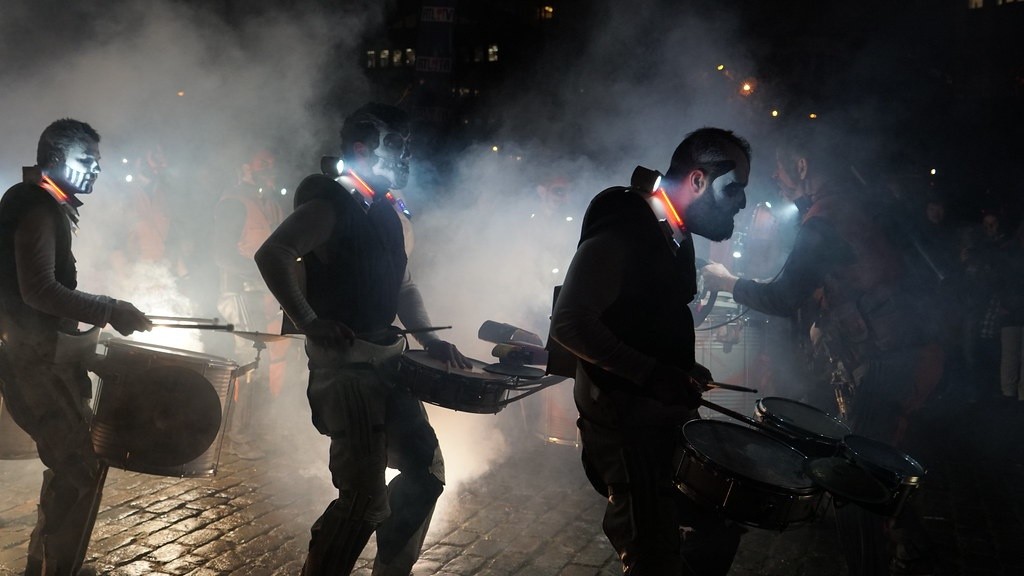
[701,125,918,453]
[0,117,154,576]
[546,125,754,576]
[254,103,473,576]
[214,151,291,463]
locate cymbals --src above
[221,329,304,343]
[481,363,547,380]
[116,363,223,465]
[801,456,891,507]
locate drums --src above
[90,338,239,481]
[671,419,821,532]
[748,397,852,468]
[399,349,509,414]
[841,435,928,515]
